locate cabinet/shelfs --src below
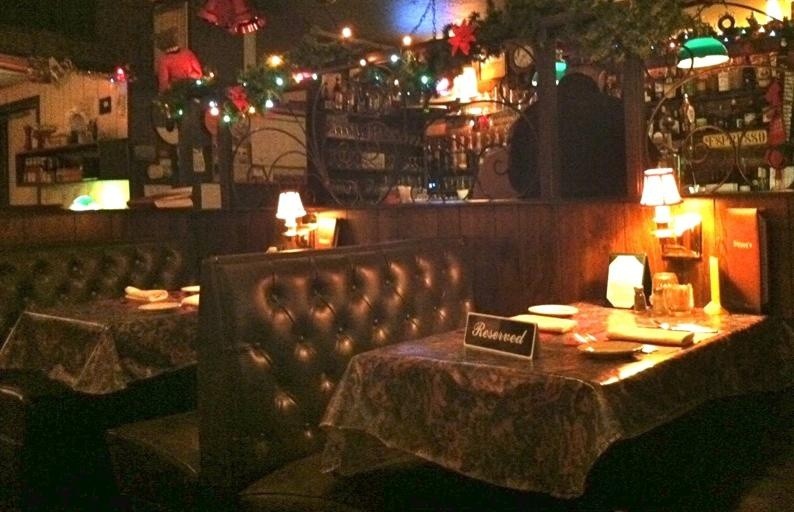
[308,18,788,196]
[14,138,130,187]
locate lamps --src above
[276,189,316,240]
[640,166,701,241]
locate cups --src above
[651,271,694,316]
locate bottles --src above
[634,286,647,314]
[22,157,52,183]
[330,72,395,117]
[382,175,470,201]
[426,125,510,174]
[648,52,777,138]
[493,80,514,112]
[757,166,769,191]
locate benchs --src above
[1,239,201,459]
[106,235,453,511]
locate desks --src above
[201,234,501,511]
[349,300,771,511]
[20,289,200,394]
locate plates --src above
[529,305,580,316]
[181,295,199,306]
[138,302,184,313]
[180,285,201,294]
[124,290,168,301]
[575,340,643,360]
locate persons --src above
[508,71,660,198]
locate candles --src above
[709,255,721,311]
